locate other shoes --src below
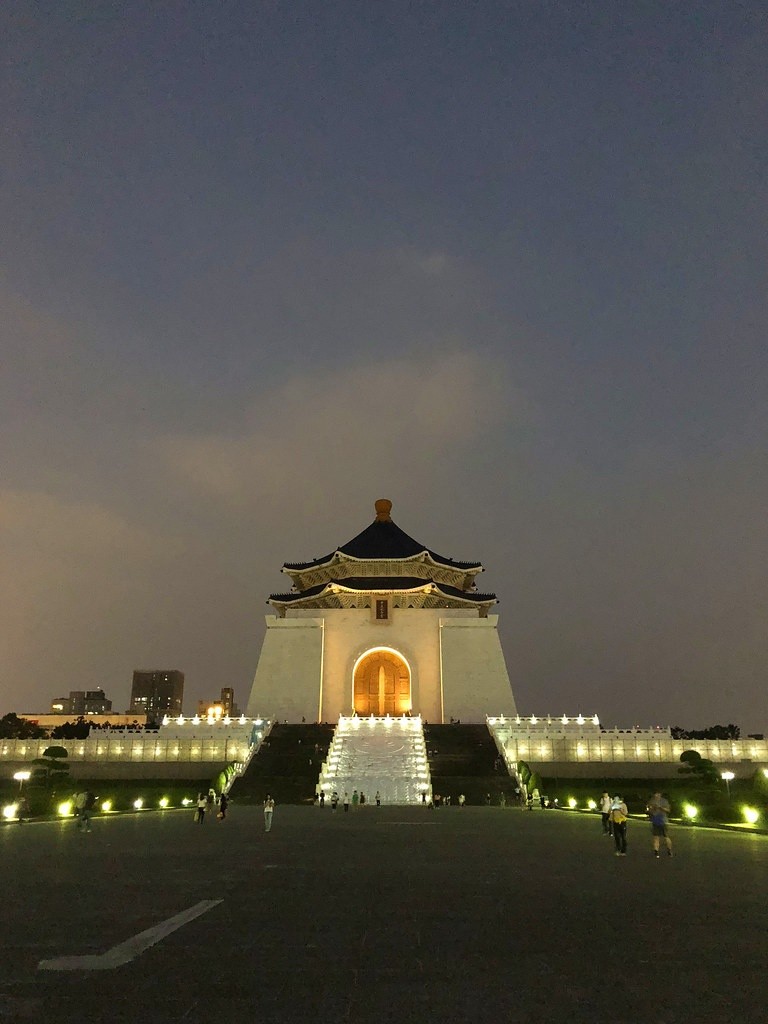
[265,829,270,832]
[609,833,613,837]
[87,829,93,832]
[603,833,607,837]
[655,851,660,858]
[615,851,626,856]
[80,830,86,833]
[668,851,673,858]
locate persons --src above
[264,794,274,832]
[425,720,431,734]
[428,749,438,757]
[207,791,214,815]
[219,793,227,824]
[331,792,339,813]
[197,794,207,824]
[422,790,466,811]
[599,791,628,856]
[268,739,271,747]
[450,717,460,726]
[487,794,491,806]
[499,792,506,809]
[315,790,325,807]
[226,793,230,807]
[514,786,545,811]
[647,790,674,858]
[375,791,381,805]
[352,790,359,813]
[343,793,350,812]
[494,753,501,771]
[360,792,366,808]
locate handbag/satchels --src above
[194,812,199,823]
[217,812,223,817]
